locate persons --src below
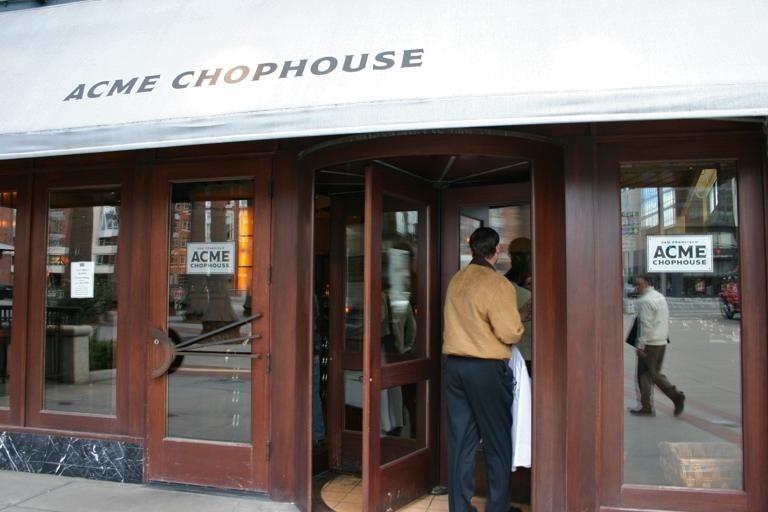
[441,227,525,512]
[518,272,531,290]
[629,275,686,418]
[313,293,325,453]
[379,274,417,436]
[503,237,531,375]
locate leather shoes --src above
[630,409,656,417]
[674,393,684,415]
